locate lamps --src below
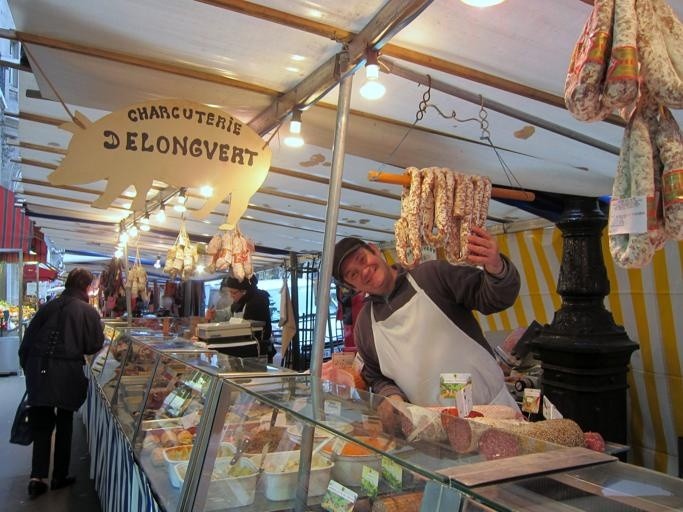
[109,0,511,259]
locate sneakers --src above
[27,480,48,496]
[50,475,75,491]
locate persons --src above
[17,264,107,498]
[206,271,278,364]
[331,222,527,428]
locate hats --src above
[332,237,363,280]
[228,278,250,289]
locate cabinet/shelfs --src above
[80,315,681,511]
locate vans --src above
[204,276,345,362]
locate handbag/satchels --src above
[9,389,33,446]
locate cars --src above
[328,283,338,307]
[44,284,64,299]
